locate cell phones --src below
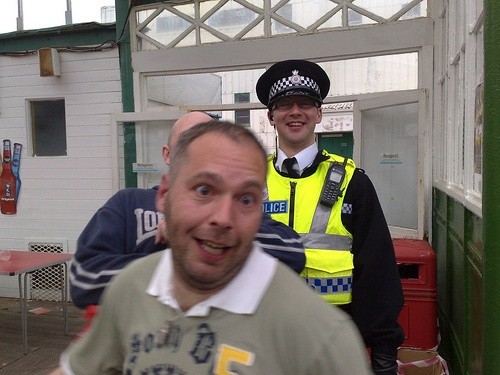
[320,157,348,207]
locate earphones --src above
[270,115,273,121]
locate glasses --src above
[271,97,319,109]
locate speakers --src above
[38,48,61,77]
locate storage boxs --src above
[398,349,443,375]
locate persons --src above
[51,121,374,375]
[255,59,403,375]
[71,112,305,309]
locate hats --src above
[255,60,331,109]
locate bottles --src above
[0,139,21,215]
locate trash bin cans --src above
[391,237,441,353]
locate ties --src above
[282,157,300,178]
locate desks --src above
[0,249,74,355]
